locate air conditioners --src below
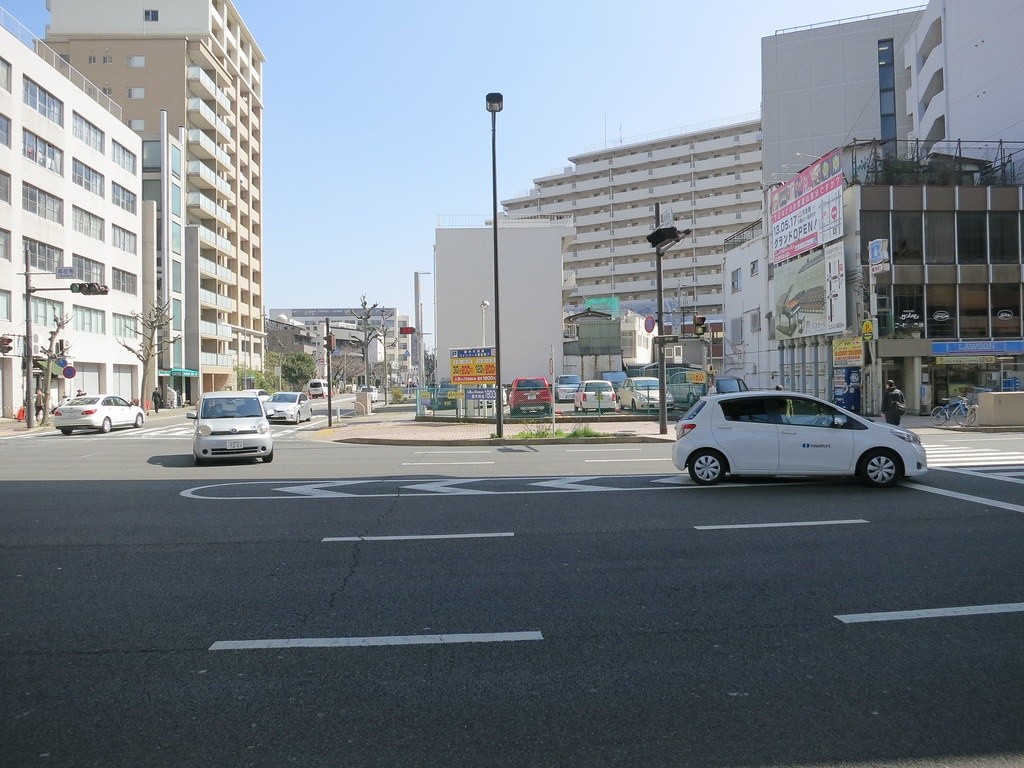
[31,334,39,356]
[56,340,72,357]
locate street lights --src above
[485,93,503,439]
[646,202,680,435]
[480,301,489,419]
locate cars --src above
[671,390,929,488]
[53,395,146,436]
[307,379,328,398]
[261,392,313,424]
[407,382,418,388]
[432,378,458,410]
[427,383,439,393]
[359,386,378,403]
[243,389,270,405]
[475,371,750,418]
[186,390,274,465]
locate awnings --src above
[37,360,65,375]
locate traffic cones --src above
[17,407,23,420]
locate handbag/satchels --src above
[890,400,907,416]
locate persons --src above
[74,390,88,398]
[705,379,718,397]
[35,385,46,421]
[877,378,910,425]
[212,402,225,417]
[127,397,140,405]
[152,387,163,412]
[775,384,793,417]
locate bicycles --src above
[931,395,976,427]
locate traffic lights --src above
[0,337,13,353]
[693,316,707,335]
[70,283,99,293]
[323,333,337,353]
